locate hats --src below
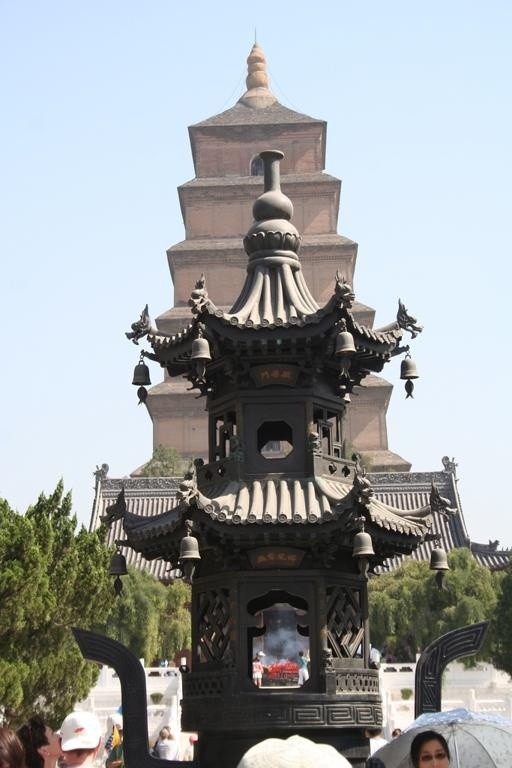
[257,651,265,657]
[59,711,101,751]
[237,734,353,768]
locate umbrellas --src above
[367,720,512,768]
[402,708,512,734]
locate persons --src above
[0,712,198,768]
[298,651,310,685]
[252,657,264,688]
[411,731,450,768]
[369,644,382,668]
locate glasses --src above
[420,752,447,761]
